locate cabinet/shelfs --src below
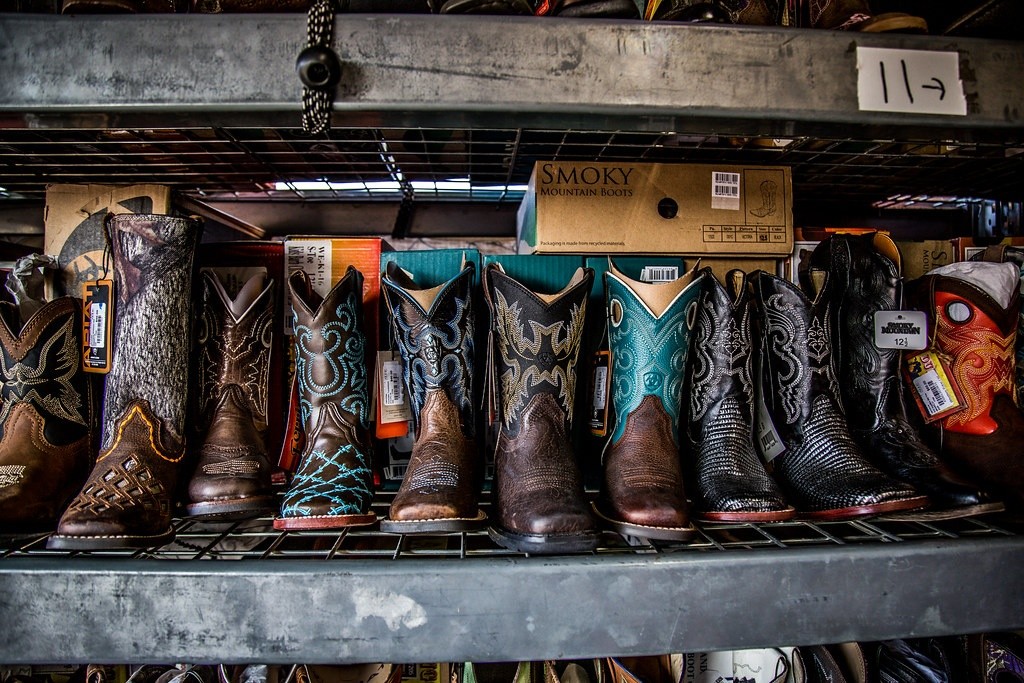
[0,6,1024,667]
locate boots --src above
[480,264,598,553]
[746,269,927,519]
[590,272,705,542]
[683,266,799,524]
[376,261,487,532]
[0,297,96,529]
[273,264,376,529]
[812,233,1005,522]
[45,213,203,551]
[910,265,1024,529]
[185,267,276,516]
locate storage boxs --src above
[513,160,796,256]
[481,251,583,492]
[683,258,779,286]
[897,239,955,281]
[584,256,686,349]
[379,248,481,489]
[282,233,382,486]
[38,177,269,473]
[191,239,283,476]
[948,235,1024,265]
